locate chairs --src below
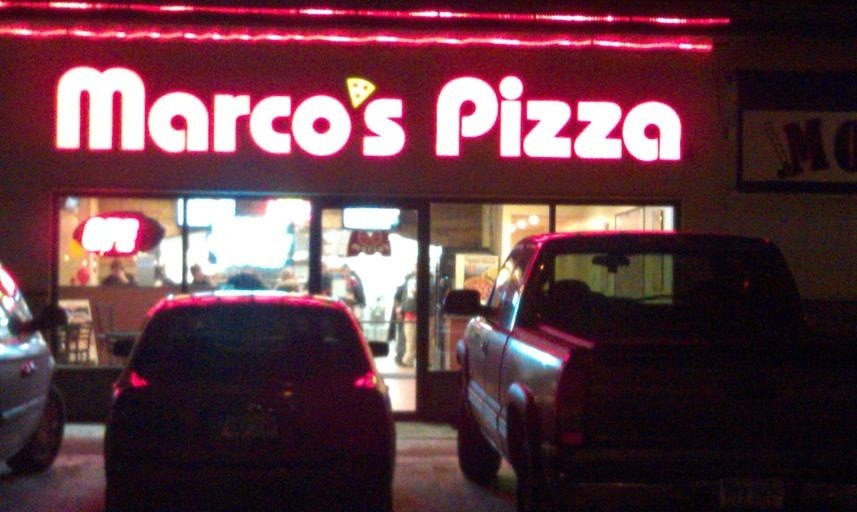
[55,302,137,365]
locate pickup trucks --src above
[442,230,856,511]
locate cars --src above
[0,261,67,477]
[103,287,396,511]
